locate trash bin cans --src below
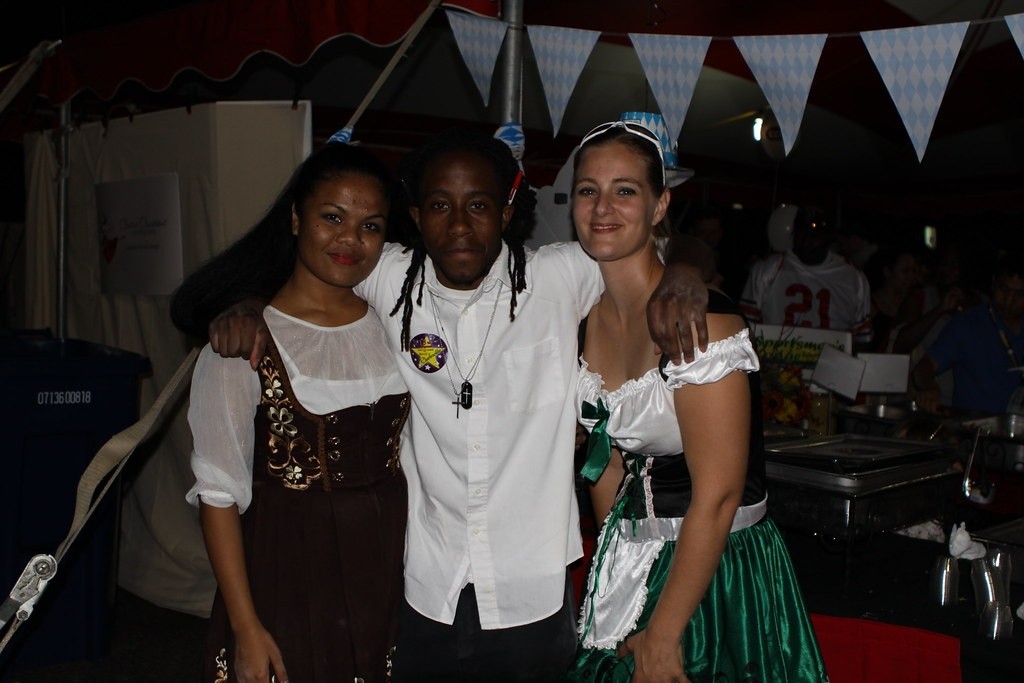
[0,327,152,668]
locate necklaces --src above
[430,282,504,418]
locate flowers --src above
[755,310,817,427]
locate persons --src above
[670,205,725,287]
[209,129,708,683]
[740,204,871,347]
[171,141,411,683]
[573,120,832,683]
[838,224,1023,414]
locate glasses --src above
[578,120,665,188]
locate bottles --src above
[807,393,832,442]
